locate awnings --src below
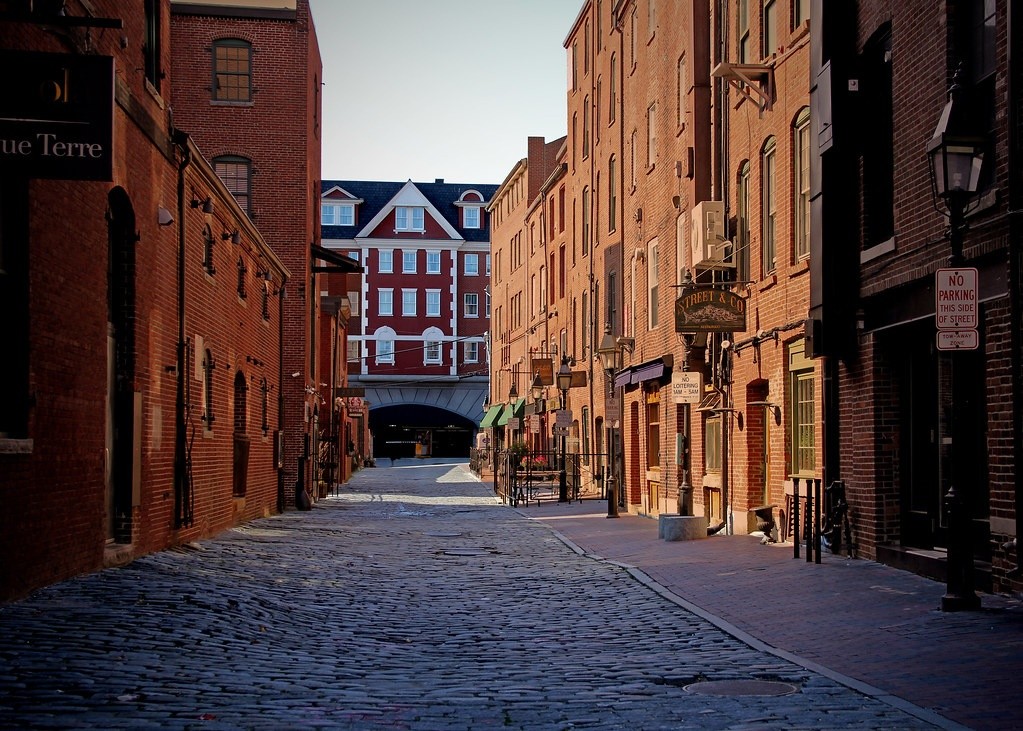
[614,366,631,388]
[479,404,503,427]
[311,244,365,274]
[497,400,524,426]
[630,353,673,385]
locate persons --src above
[389,450,393,466]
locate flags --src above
[674,288,746,332]
[532,358,553,386]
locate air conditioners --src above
[691,201,726,269]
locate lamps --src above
[275,287,288,298]
[191,197,214,213]
[256,270,272,281]
[222,229,241,245]
